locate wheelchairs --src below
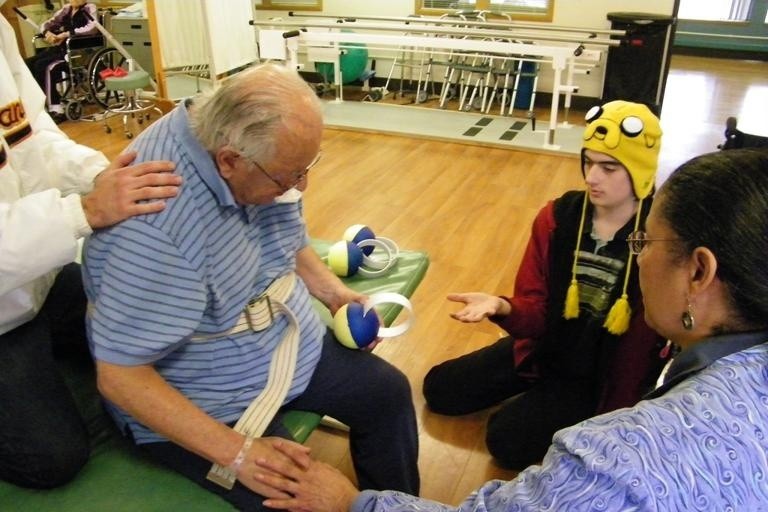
[32,8,130,122]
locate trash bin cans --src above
[600,11,676,120]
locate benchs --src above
[1,239,428,512]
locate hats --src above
[580,100,662,201]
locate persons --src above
[0,0,183,492]
[78,60,422,510]
[250,145,767,511]
[21,0,98,119]
[422,98,671,475]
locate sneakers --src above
[49,105,64,114]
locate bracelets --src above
[230,430,254,474]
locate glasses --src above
[218,131,321,193]
[626,230,688,255]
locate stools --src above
[102,70,163,139]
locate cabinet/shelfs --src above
[110,15,155,82]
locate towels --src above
[98,66,127,80]
